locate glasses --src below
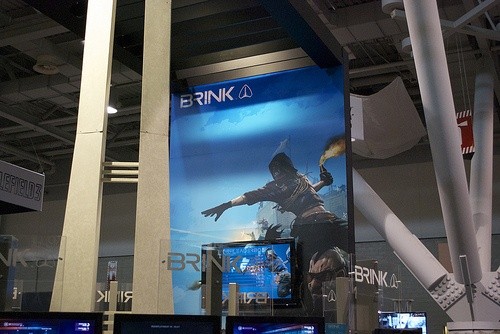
[303,265,346,284]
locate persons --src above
[244,249,292,298]
[201,152,342,319]
[306,247,351,322]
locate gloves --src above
[201,201,232,222]
[320,172,333,186]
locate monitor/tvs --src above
[0,312,102,334]
[200,238,303,311]
[113,313,221,334]
[226,316,325,334]
[378,312,428,334]
